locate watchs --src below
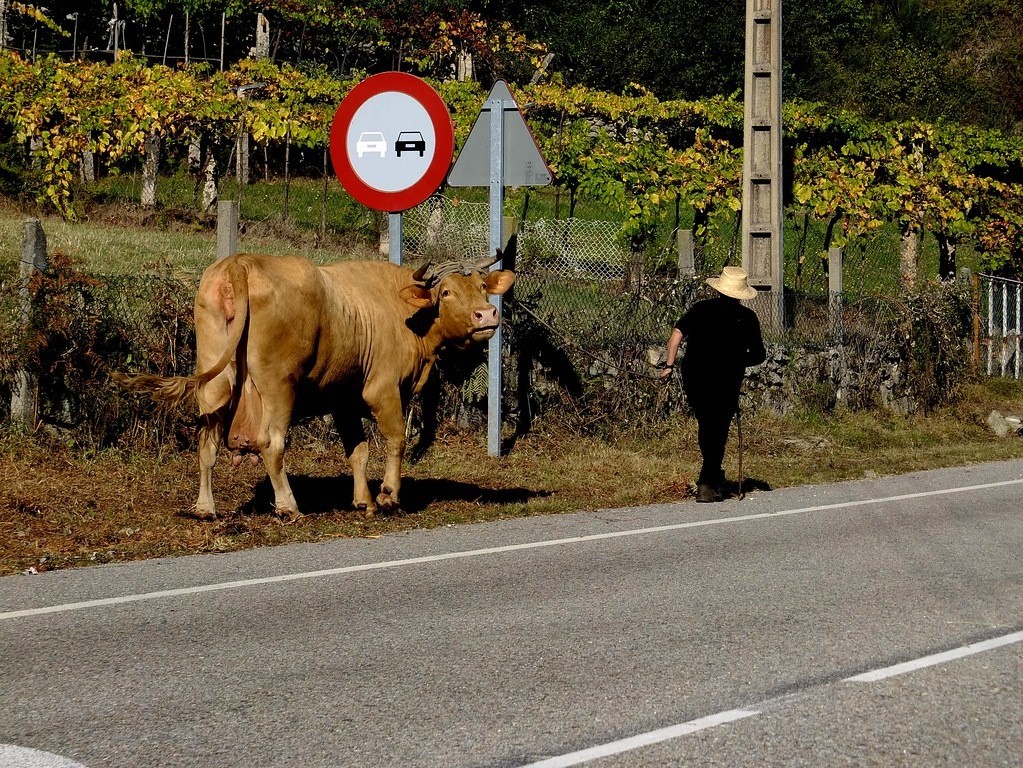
[663,364,674,370]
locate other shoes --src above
[716,472,727,486]
[696,484,714,502]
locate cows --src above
[112,247,516,520]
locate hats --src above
[706,266,758,299]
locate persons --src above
[658,265,766,503]
